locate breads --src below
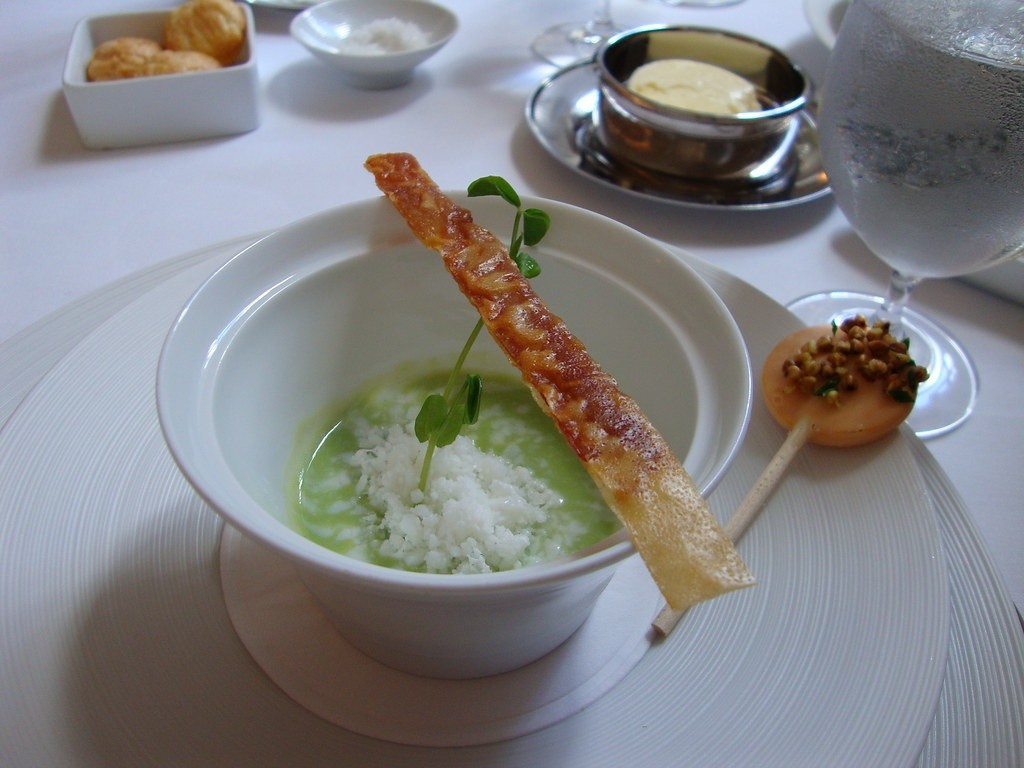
[86,0,246,83]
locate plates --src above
[526,58,832,209]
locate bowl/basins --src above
[155,190,751,680]
[597,22,812,179]
[62,2,262,149]
[289,0,459,87]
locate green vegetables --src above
[412,175,551,494]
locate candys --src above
[649,313,930,637]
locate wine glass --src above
[786,0,1024,435]
[533,1,634,68]
